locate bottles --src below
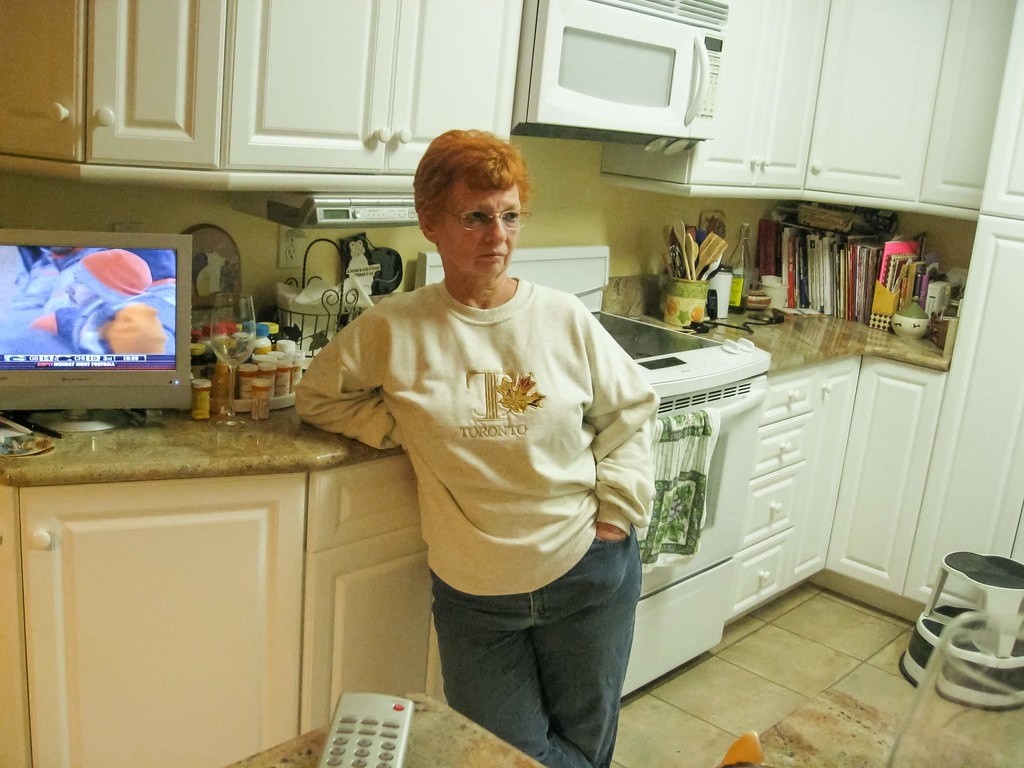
[191,343,208,379]
[259,363,277,397]
[259,322,281,351]
[725,223,754,315]
[290,360,302,394]
[191,330,201,342]
[277,360,290,396]
[237,322,272,355]
[251,379,270,420]
[226,321,236,336]
[191,379,212,421]
[214,323,227,339]
[895,296,929,339]
[201,326,213,341]
[238,363,258,400]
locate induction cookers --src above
[415,245,772,397]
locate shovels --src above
[673,220,696,279]
[695,232,728,278]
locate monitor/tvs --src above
[0,227,193,432]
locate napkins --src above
[271,276,375,370]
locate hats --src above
[74,249,152,304]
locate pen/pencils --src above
[0,411,64,439]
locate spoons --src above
[674,219,699,281]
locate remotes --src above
[317,691,415,768]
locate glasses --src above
[446,210,532,230]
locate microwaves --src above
[508,0,730,157]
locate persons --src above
[12,247,176,356]
[293,130,661,768]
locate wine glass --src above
[210,293,256,434]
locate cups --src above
[759,283,790,308]
[709,273,733,318]
[885,611,1024,768]
[277,340,296,353]
[253,351,306,365]
[664,276,709,326]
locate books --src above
[756,219,962,326]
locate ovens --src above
[620,375,768,700]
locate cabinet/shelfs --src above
[0,0,520,192]
[0,451,448,768]
[600,0,1024,624]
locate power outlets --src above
[277,224,303,267]
